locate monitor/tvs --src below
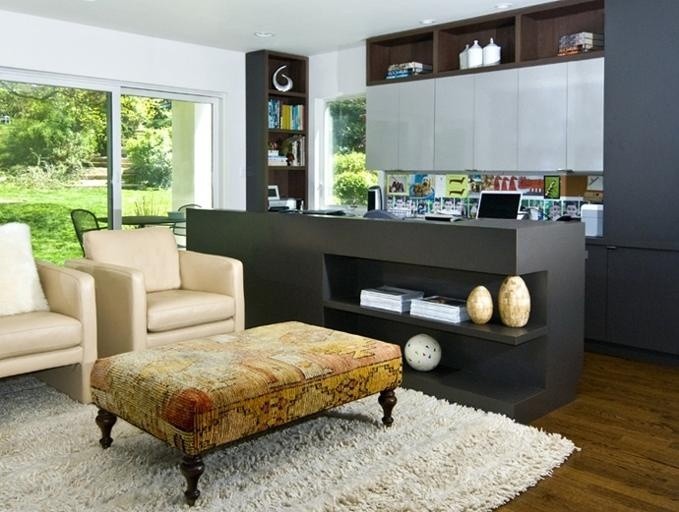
[367,188,381,213]
[475,190,522,218]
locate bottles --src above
[467,39,483,70]
[482,37,502,66]
[459,43,470,70]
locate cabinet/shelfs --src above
[321,296,548,424]
[246,49,309,212]
[584,244,679,359]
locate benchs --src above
[91,319,405,507]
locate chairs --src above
[62,229,246,359]
[0,261,99,405]
[169,202,203,250]
[70,208,108,257]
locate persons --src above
[395,197,405,210]
[443,198,453,214]
[405,198,416,215]
[471,201,477,219]
[417,202,426,214]
[564,200,578,218]
[457,199,466,217]
[547,202,562,220]
[391,181,403,192]
[431,199,441,214]
[385,196,394,212]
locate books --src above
[359,286,473,323]
[268,98,304,166]
[386,60,431,80]
[558,31,603,56]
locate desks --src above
[96,215,186,229]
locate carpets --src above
[0,374,583,512]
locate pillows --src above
[0,221,51,316]
